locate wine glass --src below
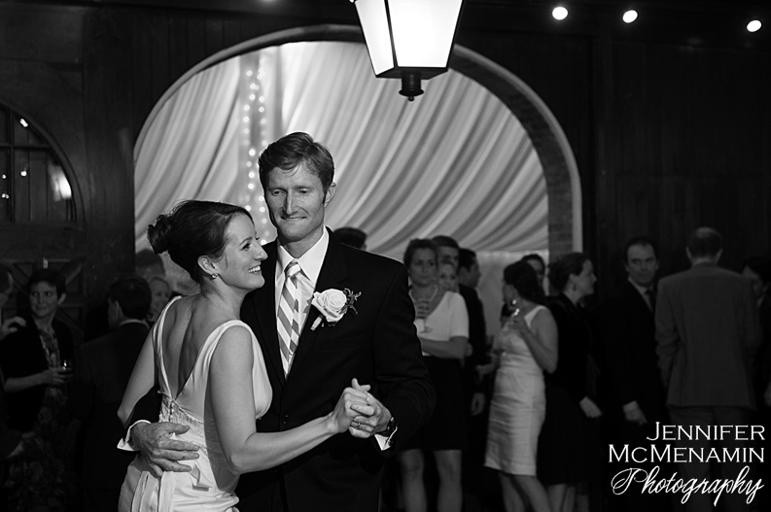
[416,291,432,336]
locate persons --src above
[396,225,770,510]
[117,133,432,511]
[336,227,364,252]
[115,202,374,512]
[0,267,179,510]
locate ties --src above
[277,261,300,379]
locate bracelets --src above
[384,414,401,437]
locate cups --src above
[49,359,69,369]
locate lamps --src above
[355,0,466,101]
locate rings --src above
[357,419,363,429]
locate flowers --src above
[310,287,358,328]
